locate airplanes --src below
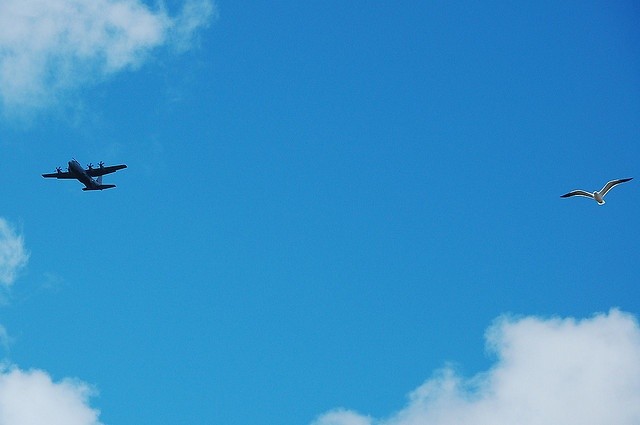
[42,158,128,190]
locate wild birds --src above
[559,177,634,205]
